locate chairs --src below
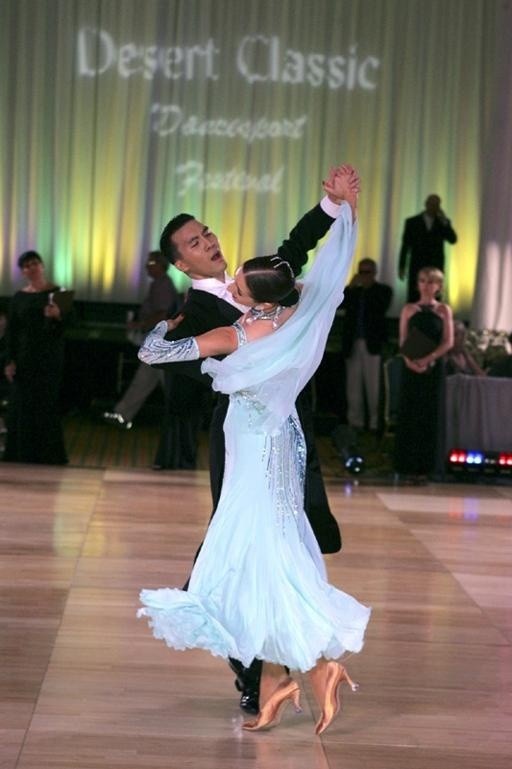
[374,353,405,463]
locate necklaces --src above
[249,306,282,322]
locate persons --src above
[446,320,483,377]
[393,268,455,485]
[113,250,182,424]
[399,194,458,304]
[2,250,75,469]
[339,258,392,437]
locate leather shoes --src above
[235,679,259,714]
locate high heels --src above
[313,661,360,736]
[240,677,304,731]
